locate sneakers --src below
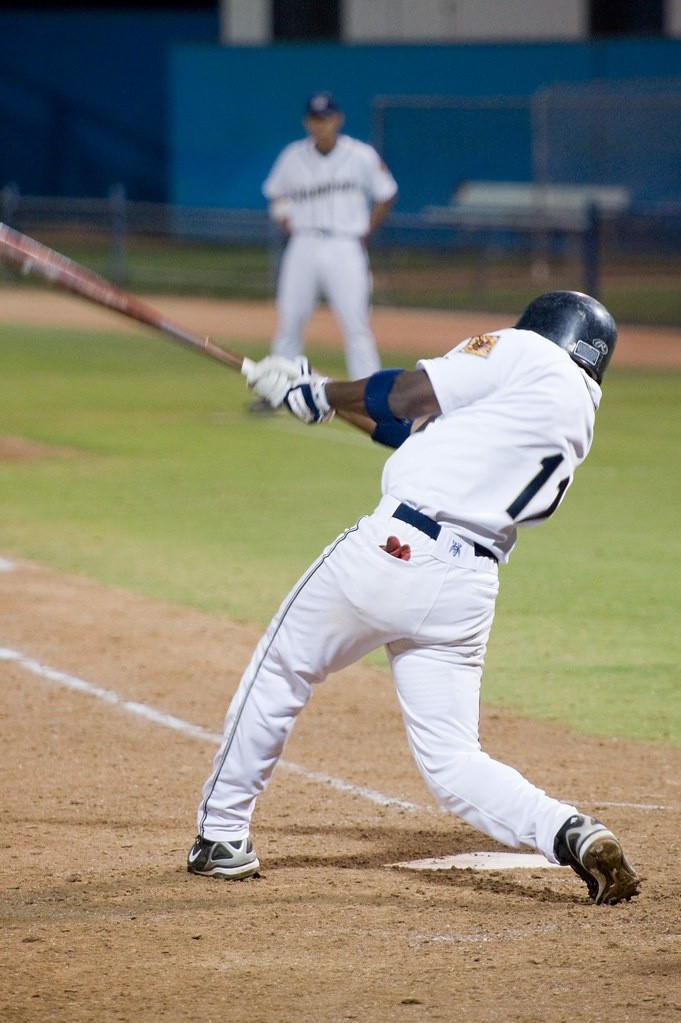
[554,814,641,904]
[187,835,259,881]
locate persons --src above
[248,92,399,414]
[187,290,644,909]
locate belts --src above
[393,504,499,564]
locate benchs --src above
[423,180,634,282]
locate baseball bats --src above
[0,222,253,379]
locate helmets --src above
[515,290,616,385]
[304,92,339,118]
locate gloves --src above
[246,356,336,422]
